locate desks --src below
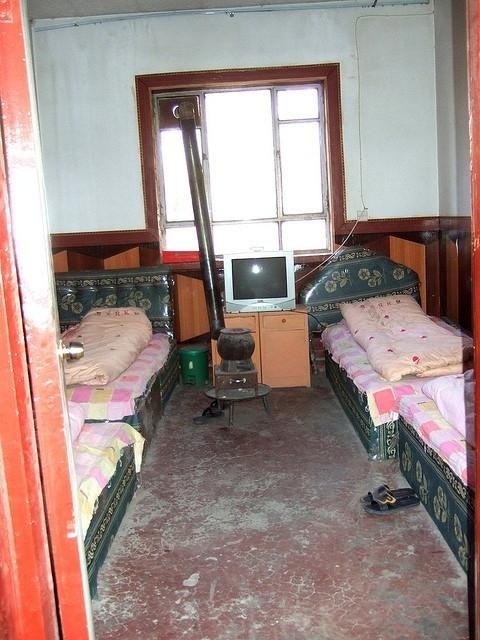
[202,384,272,427]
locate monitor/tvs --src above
[224,250,295,313]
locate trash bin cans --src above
[180,344,209,386]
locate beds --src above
[324,314,474,574]
[68,422,146,600]
[54,267,181,461]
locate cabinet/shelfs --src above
[212,311,309,392]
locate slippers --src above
[193,400,229,425]
[360,484,421,514]
